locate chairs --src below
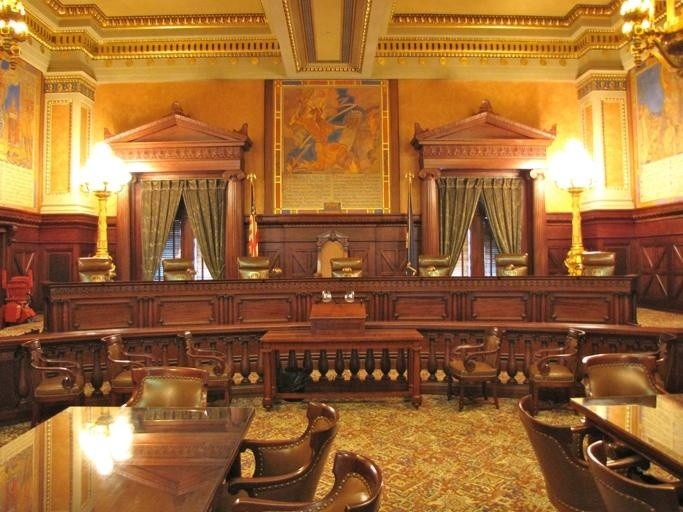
[1,332,235,428]
[580,250,618,278]
[76,257,112,283]
[161,257,198,280]
[447,326,503,412]
[519,328,681,510]
[310,227,351,277]
[328,256,362,278]
[416,253,452,278]
[492,250,530,277]
[217,402,383,511]
[233,254,272,279]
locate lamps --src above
[0,0,29,71]
[542,134,594,276]
[617,0,682,82]
[73,141,133,273]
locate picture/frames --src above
[260,80,402,215]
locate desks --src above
[36,273,642,328]
[252,322,428,412]
[2,406,256,510]
